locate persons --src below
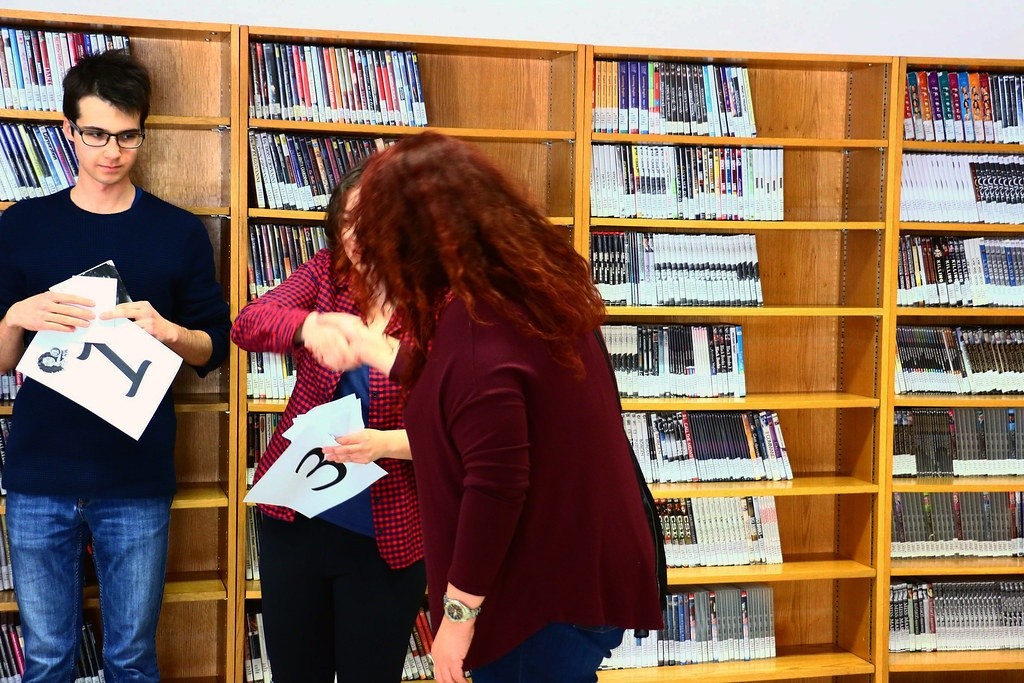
[305,132,663,683]
[0,50,229,683]
[233,173,427,683]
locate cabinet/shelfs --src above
[0,8,1023,681]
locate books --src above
[0,370,110,683]
[888,70,1024,653]
[247,43,428,488]
[0,27,138,202]
[243,609,274,683]
[591,60,790,671]
[246,507,265,581]
[401,605,470,680]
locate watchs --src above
[444,593,481,623]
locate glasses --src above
[68,119,146,149]
